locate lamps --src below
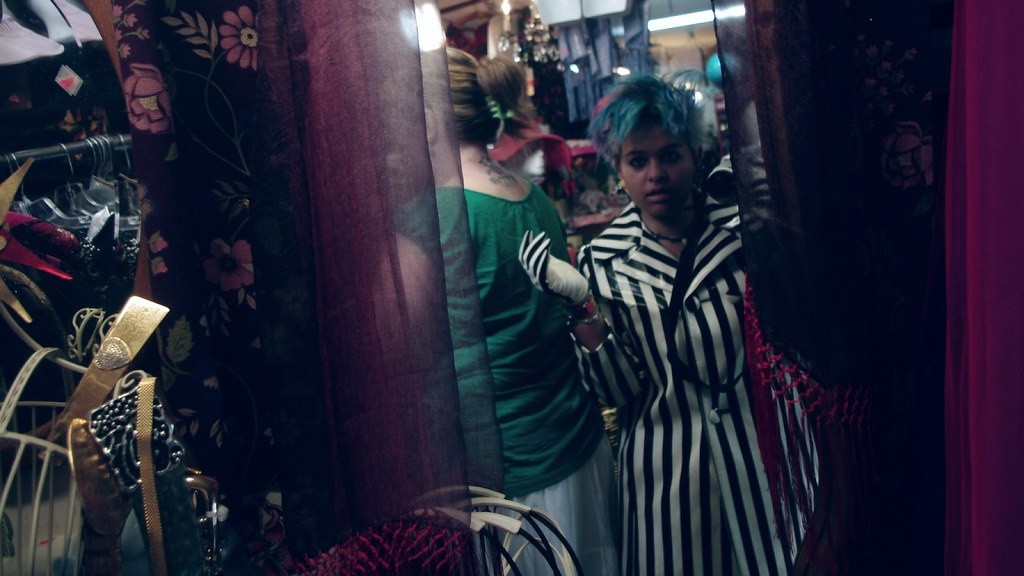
[498,0,552,63]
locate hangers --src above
[0,129,141,232]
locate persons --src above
[386,47,824,576]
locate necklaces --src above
[641,226,701,243]
